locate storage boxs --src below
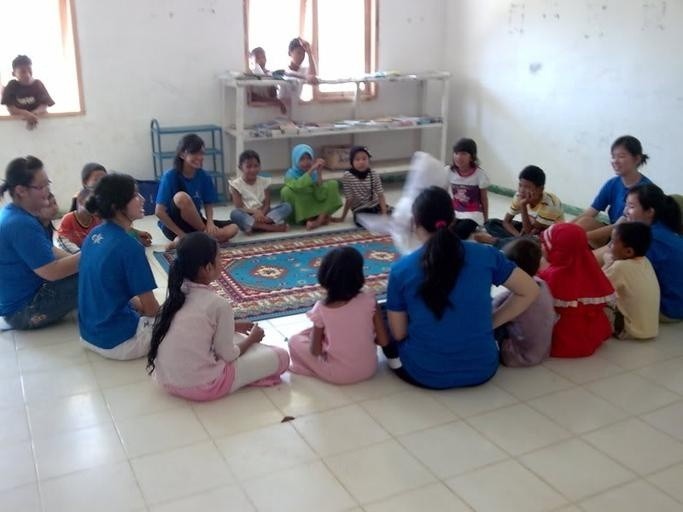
[322,144,354,170]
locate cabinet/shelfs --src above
[220,71,450,201]
[151,119,227,198]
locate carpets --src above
[153,229,403,322]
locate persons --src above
[2,154,161,362]
[231,148,291,235]
[290,246,390,384]
[280,142,342,229]
[444,137,490,239]
[330,141,394,227]
[0,54,55,130]
[147,232,289,401]
[381,181,682,389]
[475,163,565,250]
[249,46,288,114]
[278,35,317,100]
[155,136,239,252]
[572,136,652,247]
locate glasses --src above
[22,179,53,191]
